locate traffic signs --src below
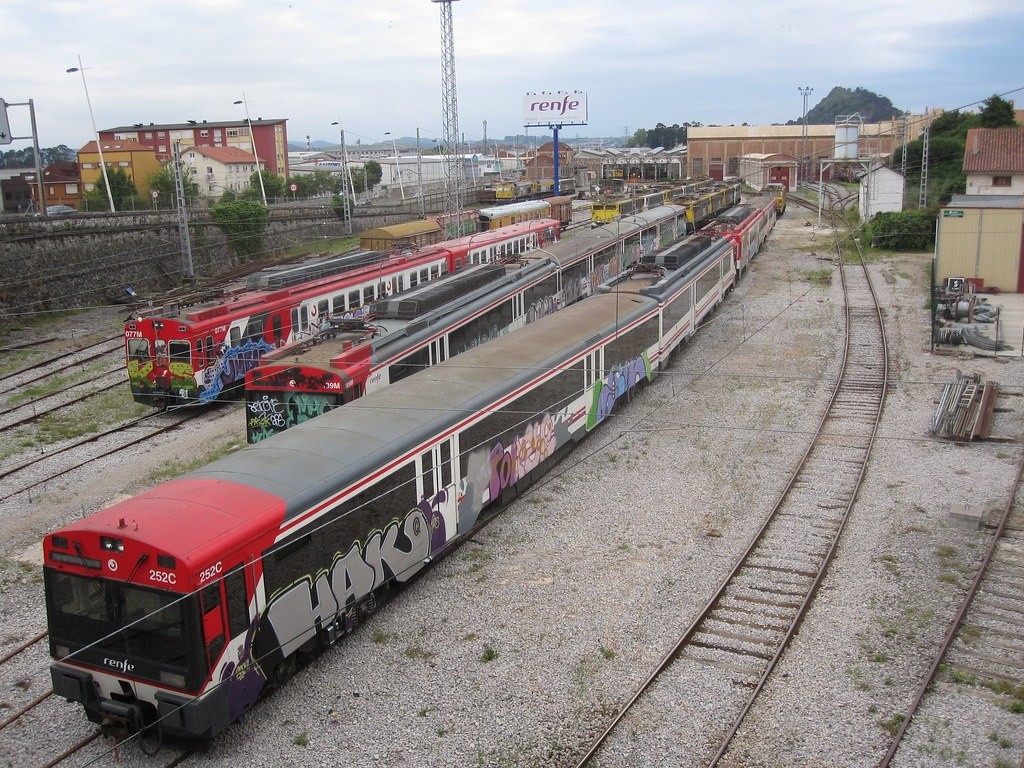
[0,99,13,146]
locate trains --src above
[662,178,742,236]
[40,193,778,756]
[244,204,689,445]
[587,175,717,228]
[758,182,788,216]
[120,216,562,414]
[494,176,577,204]
[357,193,574,253]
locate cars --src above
[34,205,78,216]
[355,198,372,206]
[412,192,425,199]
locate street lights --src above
[331,114,356,205]
[464,139,476,186]
[803,88,815,193]
[384,127,406,199]
[431,136,447,190]
[797,86,809,191]
[233,90,268,207]
[491,139,502,180]
[65,52,116,213]
[510,142,519,180]
